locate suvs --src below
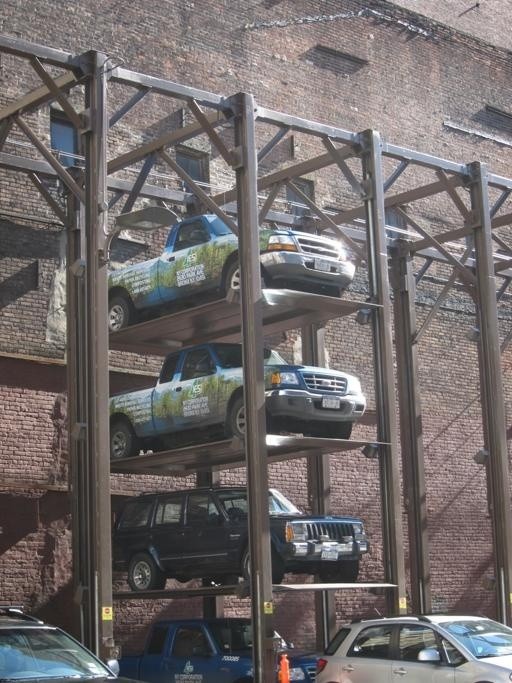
[0,605,136,682]
[112,486,371,592]
[316,613,511,682]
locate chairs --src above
[186,503,247,523]
[179,229,208,246]
[185,361,209,379]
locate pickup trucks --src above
[108,341,367,459]
[108,214,356,332]
[102,618,322,682]
